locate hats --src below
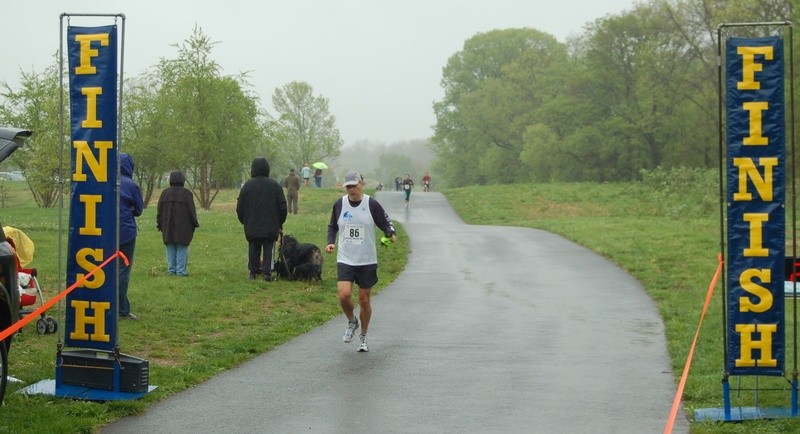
[305,163,307,165]
[343,170,361,187]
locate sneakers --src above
[357,335,368,352]
[343,316,359,342]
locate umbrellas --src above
[312,162,329,170]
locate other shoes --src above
[264,276,272,281]
[249,273,256,279]
[121,313,136,320]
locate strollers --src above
[2,226,58,334]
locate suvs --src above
[0,127,32,405]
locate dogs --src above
[274,234,324,282]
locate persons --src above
[157,171,200,276]
[375,175,403,191]
[325,171,397,352]
[119,153,143,318]
[401,174,414,204]
[422,173,431,192]
[285,167,300,214]
[236,158,287,283]
[300,162,312,187]
[312,169,324,188]
